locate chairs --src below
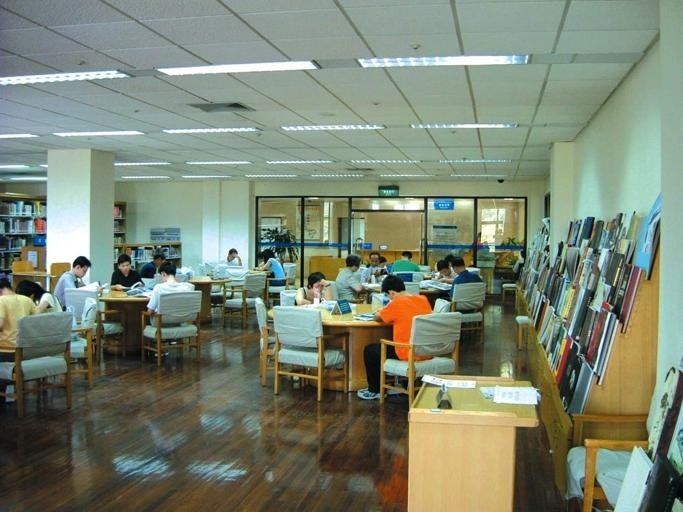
[565,366,683,512]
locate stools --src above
[502,284,517,300]
[516,315,530,350]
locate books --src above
[519,196,659,412]
[0,200,46,282]
[353,313,377,321]
[113,206,180,271]
[123,281,142,289]
[79,281,109,290]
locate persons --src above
[513,250,526,282]
[219,248,242,266]
[146,261,195,358]
[469,233,490,255]
[357,275,434,401]
[15,279,64,314]
[54,255,102,311]
[293,271,334,308]
[0,278,39,409]
[110,254,145,291]
[335,251,485,303]
[255,249,287,286]
[140,253,166,278]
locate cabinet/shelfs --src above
[114,201,128,263]
[0,193,47,271]
[114,242,182,268]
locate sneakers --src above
[5,391,15,402]
[358,388,387,400]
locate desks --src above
[407,376,539,512]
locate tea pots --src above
[369,292,390,315]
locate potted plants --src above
[261,227,300,277]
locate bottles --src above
[313,284,319,307]
[370,275,375,283]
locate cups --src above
[102,282,109,294]
